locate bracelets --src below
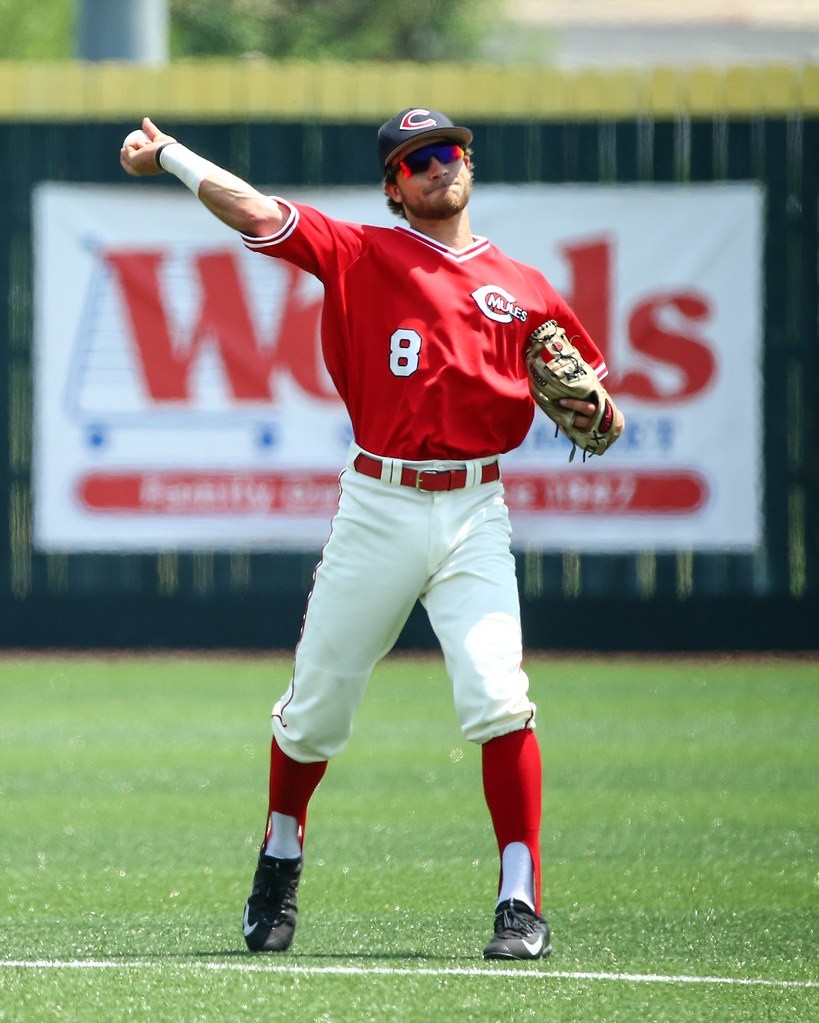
[155,141,175,169]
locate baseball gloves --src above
[525,321,625,455]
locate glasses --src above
[389,140,466,186]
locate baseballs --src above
[123,129,151,148]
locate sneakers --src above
[241,842,303,952]
[483,898,551,960]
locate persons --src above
[120,109,624,960]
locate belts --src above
[355,454,500,496]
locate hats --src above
[377,107,473,171]
